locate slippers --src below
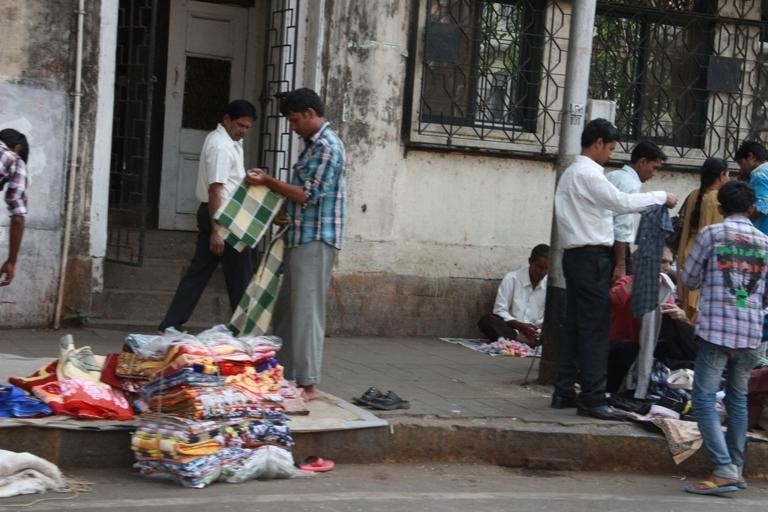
[681,477,743,498]
[297,454,336,472]
[350,385,412,411]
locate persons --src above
[155,96,259,340]
[242,86,347,403]
[0,125,32,287]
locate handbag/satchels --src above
[664,213,684,253]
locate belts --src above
[562,244,611,257]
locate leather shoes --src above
[577,400,628,423]
[549,390,582,410]
